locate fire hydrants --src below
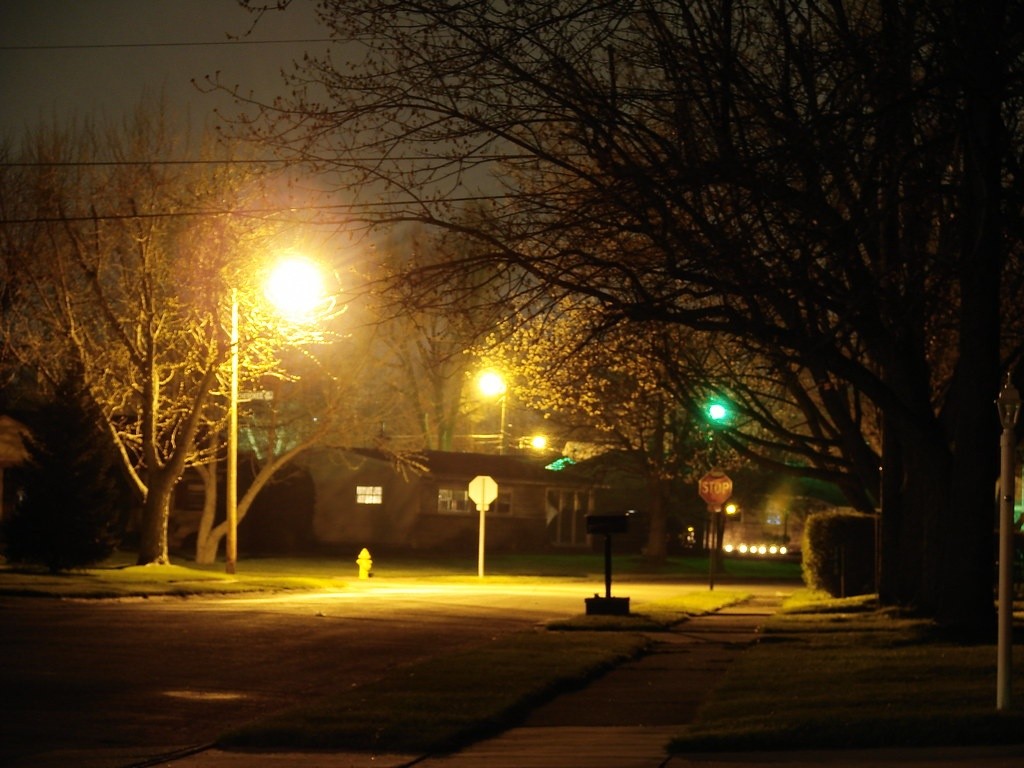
[357,547,374,579]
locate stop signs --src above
[698,468,737,506]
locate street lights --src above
[226,260,329,575]
[996,372,1024,719]
[484,370,510,456]
